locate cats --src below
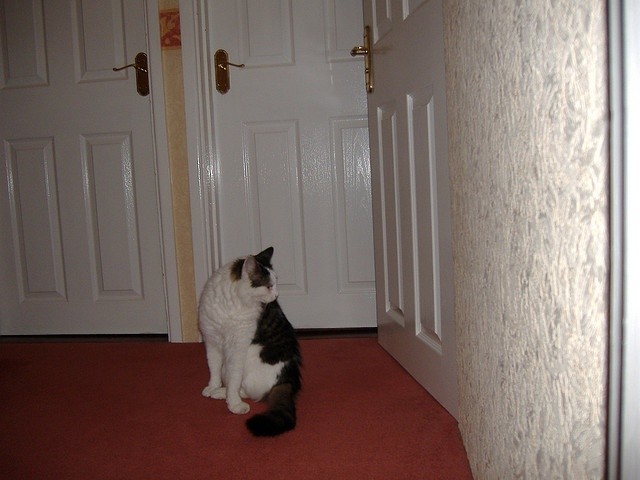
[196,245,303,438]
